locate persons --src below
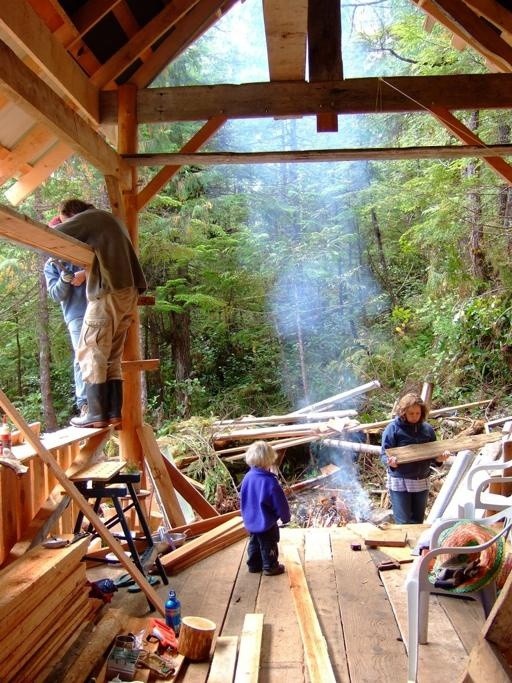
[42,213,88,418]
[378,391,454,524]
[47,198,149,428]
[239,438,291,576]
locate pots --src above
[152,528,192,555]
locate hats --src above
[426,519,507,595]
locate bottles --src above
[0,414,11,451]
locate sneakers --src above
[249,564,285,576]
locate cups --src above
[164,590,182,637]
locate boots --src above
[69,378,123,428]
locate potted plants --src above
[122,457,143,494]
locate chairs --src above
[404,508,512,683]
[457,460,512,519]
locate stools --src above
[61,471,169,613]
[120,490,151,533]
